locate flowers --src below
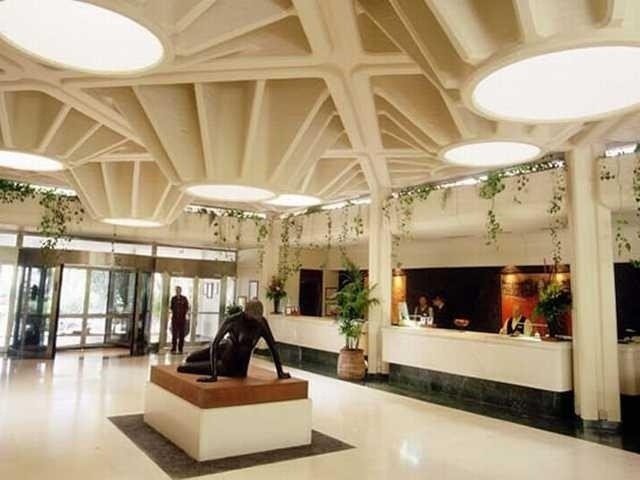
[264,274,289,303]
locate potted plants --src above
[530,257,571,342]
[325,245,382,383]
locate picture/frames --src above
[325,286,338,316]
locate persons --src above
[428,294,455,328]
[413,296,434,322]
[170,286,189,353]
[498,304,533,337]
[176,299,291,382]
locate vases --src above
[270,297,283,314]
